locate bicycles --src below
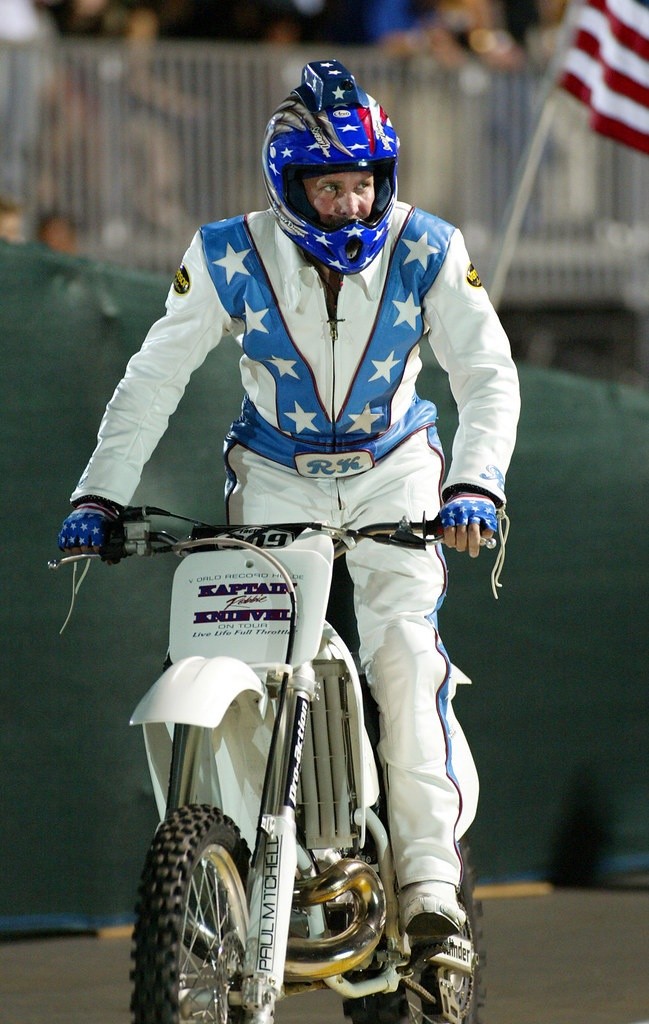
[43,501,486,1024]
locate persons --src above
[57,61,521,939]
[0,0,649,252]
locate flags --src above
[556,0,649,156]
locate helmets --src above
[260,59,400,276]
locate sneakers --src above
[399,882,466,936]
